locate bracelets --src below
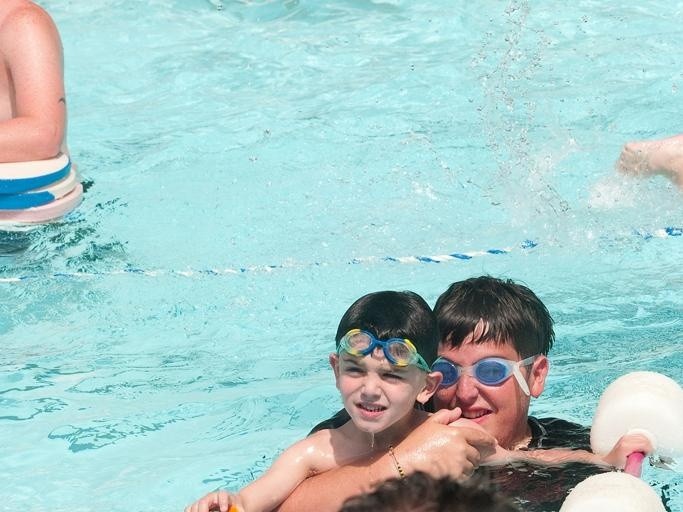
[382,441,407,486]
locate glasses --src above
[431,353,540,386]
[334,328,429,375]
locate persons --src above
[613,133,681,192]
[0,1,73,266]
[273,273,626,510]
[180,289,655,511]
[336,466,533,512]
